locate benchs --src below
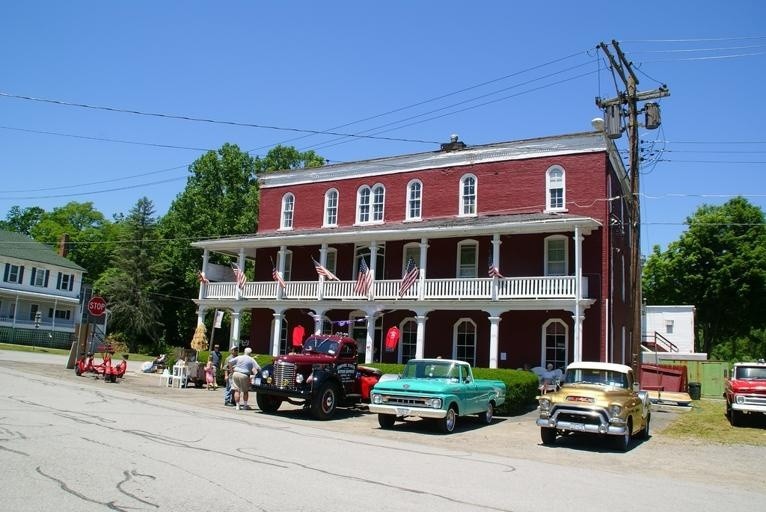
[516,366,562,394]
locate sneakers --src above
[225,401,249,411]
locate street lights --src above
[590,117,640,239]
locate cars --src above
[535,362,651,450]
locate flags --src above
[354,256,373,296]
[398,255,419,297]
[487,254,504,279]
[312,256,341,283]
[230,261,246,288]
[269,257,287,288]
[197,270,209,284]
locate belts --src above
[234,371,250,378]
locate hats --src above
[215,345,219,348]
[231,346,239,351]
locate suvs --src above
[723,359,766,426]
[249,335,381,420]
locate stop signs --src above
[88,297,107,316]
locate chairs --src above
[160,365,189,389]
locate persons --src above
[537,363,556,395]
[523,362,534,372]
[210,344,223,389]
[228,347,262,411]
[203,354,217,391]
[222,345,240,405]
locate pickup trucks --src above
[369,356,506,434]
